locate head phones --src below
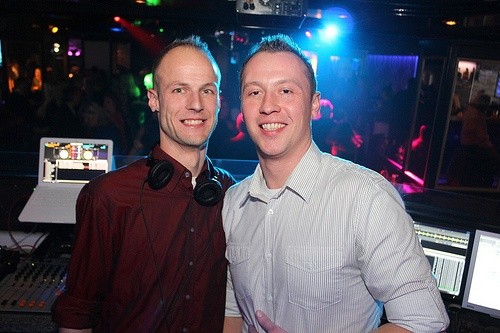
[145,147,225,207]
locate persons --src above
[452,69,500,158]
[313,99,361,158]
[52,36,236,333]
[0,62,158,167]
[207,95,259,173]
[221,36,450,333]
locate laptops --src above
[19,138,114,224]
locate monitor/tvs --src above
[411,215,500,326]
